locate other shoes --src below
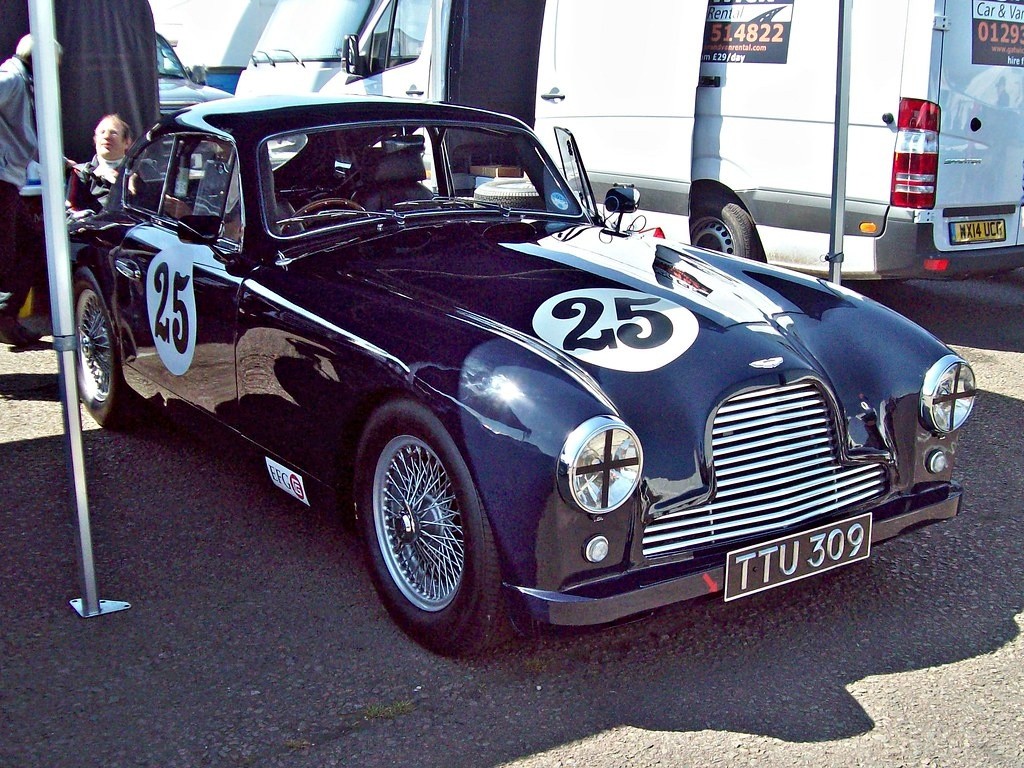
[0,317,43,345]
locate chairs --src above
[349,154,436,213]
[192,163,307,237]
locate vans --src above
[314,0,1024,281]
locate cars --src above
[52,93,979,659]
[155,31,235,173]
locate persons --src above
[64,113,166,216]
[0,34,65,344]
